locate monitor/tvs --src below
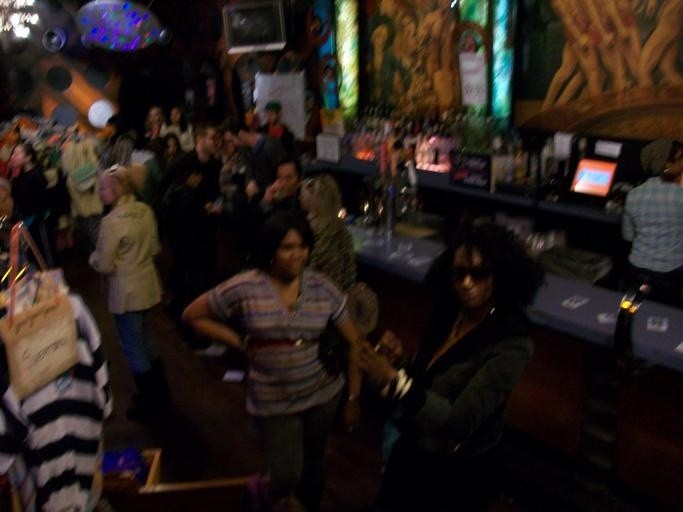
[222,0,287,55]
[568,158,618,197]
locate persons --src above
[1,97,380,511]
[350,216,547,511]
[198,0,682,124]
[621,138,682,311]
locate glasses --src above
[455,267,488,280]
[108,163,118,176]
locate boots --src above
[126,361,175,421]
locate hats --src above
[640,139,672,177]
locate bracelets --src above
[381,367,416,403]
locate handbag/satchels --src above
[0,223,80,399]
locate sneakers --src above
[194,343,227,356]
